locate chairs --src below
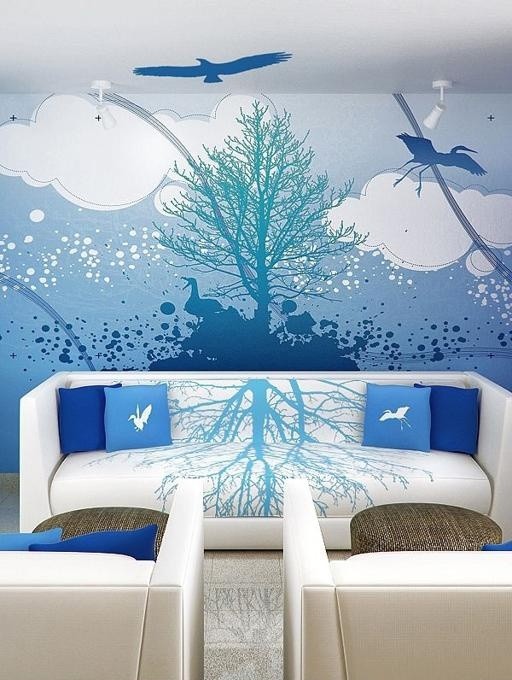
[279,477,510,679]
[1,479,207,680]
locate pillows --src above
[2,527,63,551]
[53,383,173,456]
[363,383,481,455]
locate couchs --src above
[16,370,512,550]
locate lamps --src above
[421,80,454,131]
[91,80,118,135]
